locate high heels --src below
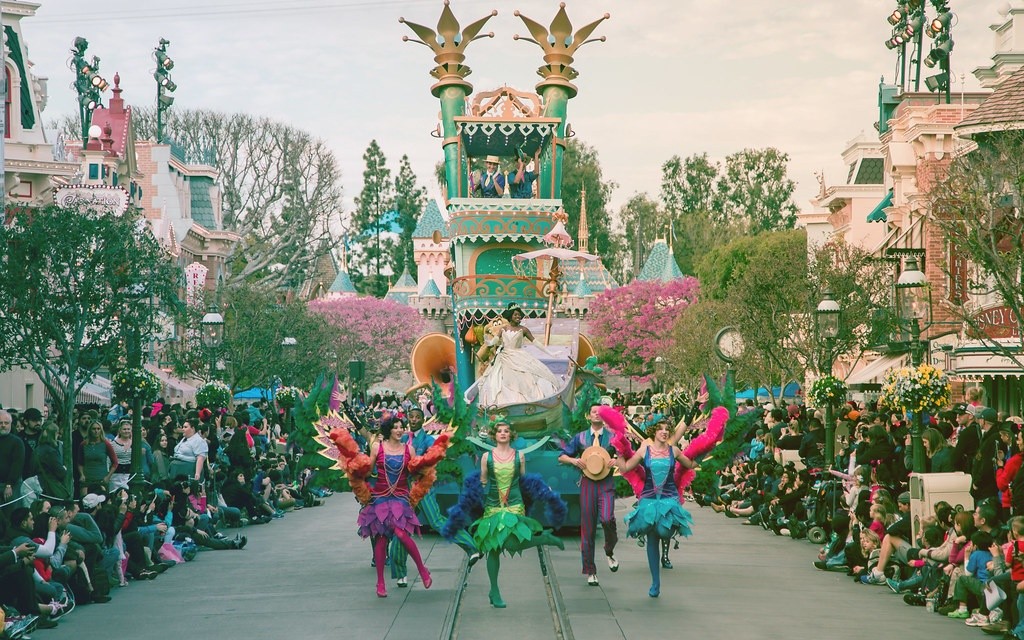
[373,581,388,597]
[541,528,565,551]
[420,567,433,589]
[487,592,507,608]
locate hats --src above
[639,413,674,431]
[581,445,613,481]
[23,407,45,420]
[847,400,858,410]
[976,406,998,423]
[83,493,105,510]
[844,410,861,422]
[762,403,775,412]
[756,429,764,437]
[953,403,976,418]
[786,404,800,419]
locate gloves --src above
[532,338,562,359]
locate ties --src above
[593,432,600,447]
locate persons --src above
[389,408,479,588]
[478,303,563,406]
[0,398,330,640]
[332,389,391,567]
[557,404,626,587]
[605,386,1024,639]
[357,414,432,597]
[468,423,565,607]
[469,155,505,198]
[507,145,542,199]
[352,389,412,420]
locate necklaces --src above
[386,447,402,452]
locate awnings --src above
[867,192,893,223]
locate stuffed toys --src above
[477,317,510,378]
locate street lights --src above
[816,291,842,470]
[199,301,225,382]
[893,254,929,473]
[281,335,298,435]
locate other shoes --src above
[608,555,619,571]
[371,555,390,567]
[587,573,599,586]
[4,482,334,640]
[397,576,407,588]
[468,551,486,566]
[648,584,661,597]
[690,475,1019,640]
[661,557,673,570]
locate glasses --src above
[28,417,43,423]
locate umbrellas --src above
[512,207,602,346]
